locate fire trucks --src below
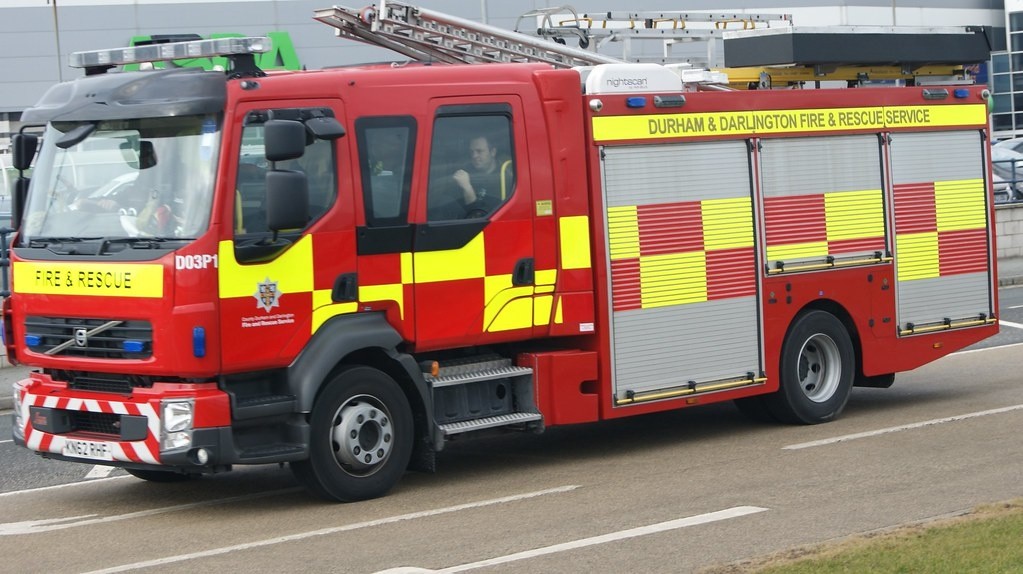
[0,0,1000,503]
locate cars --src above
[69,163,268,220]
[988,138,1023,204]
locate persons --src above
[453,134,511,216]
[99,138,181,211]
